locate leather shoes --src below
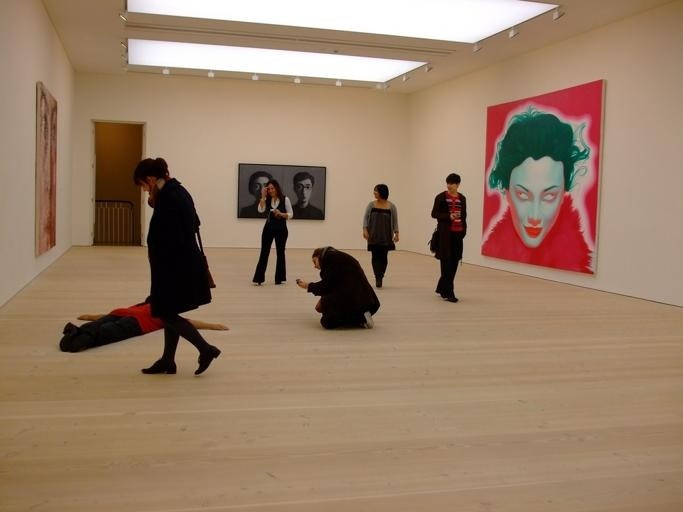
[142,360,176,374]
[195,346,220,374]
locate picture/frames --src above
[233,162,328,222]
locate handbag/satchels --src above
[430,231,440,259]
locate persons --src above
[60,296,230,353]
[363,184,400,287]
[240,172,273,218]
[296,247,380,329]
[431,174,467,303]
[481,105,595,274]
[253,179,294,285]
[134,158,220,375]
[292,172,324,220]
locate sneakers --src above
[360,311,374,328]
